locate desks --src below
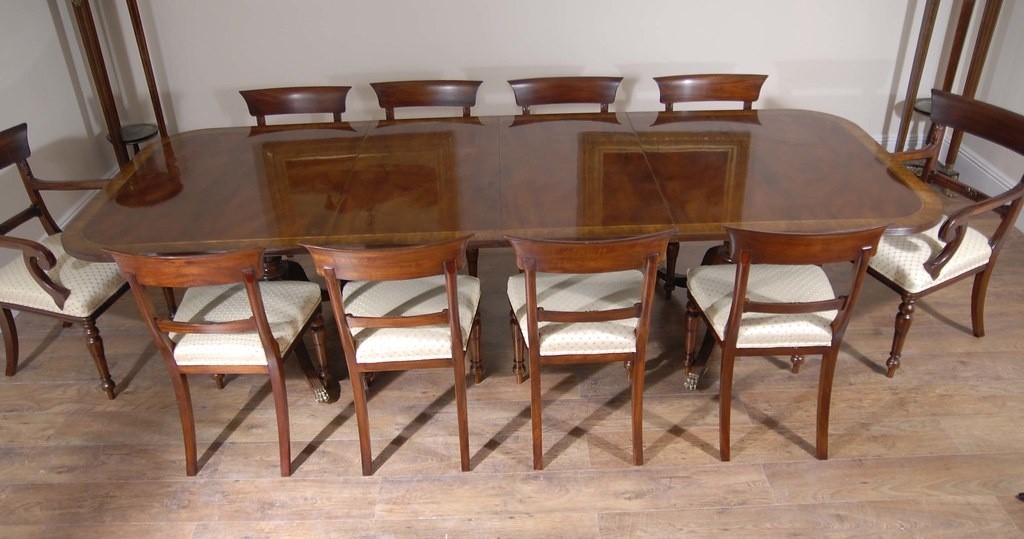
[60,107,946,406]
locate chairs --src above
[0,72,1024,477]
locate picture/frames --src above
[576,131,750,239]
[262,131,461,237]
